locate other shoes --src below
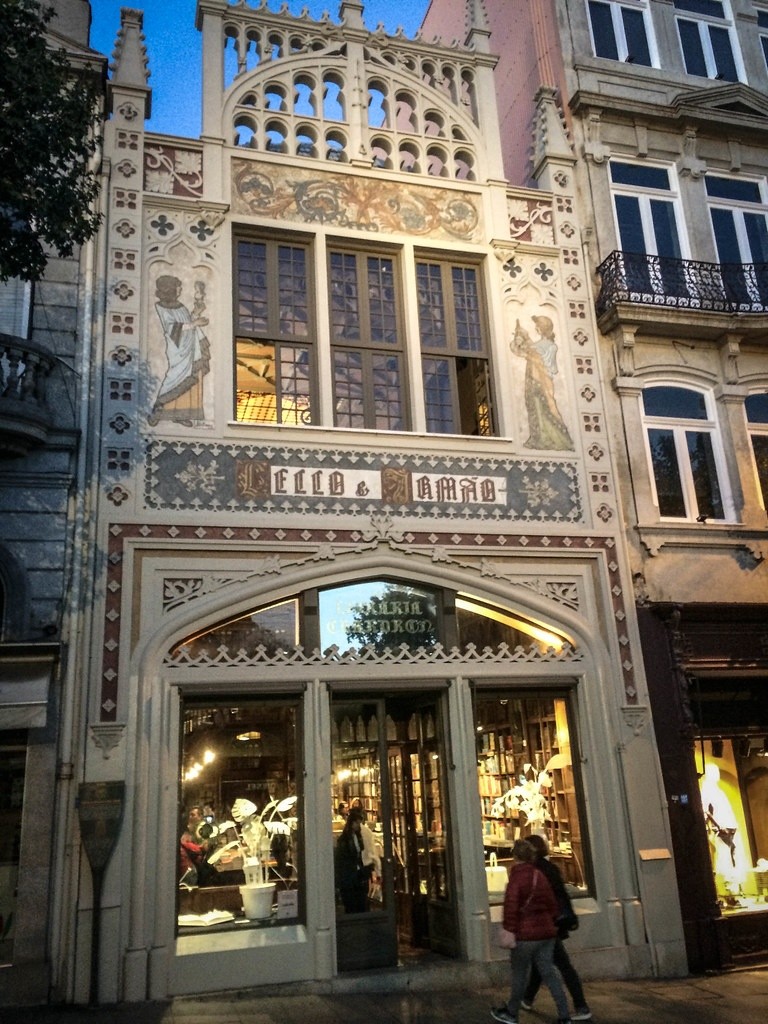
[570,1011,592,1020]
[519,1000,533,1010]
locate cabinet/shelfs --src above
[331,696,586,886]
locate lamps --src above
[236,730,260,742]
[736,740,751,758]
[763,739,768,757]
[711,739,724,757]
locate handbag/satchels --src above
[498,928,516,949]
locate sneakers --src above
[557,1019,571,1024]
[489,1007,518,1024]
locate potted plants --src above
[208,794,299,919]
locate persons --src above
[350,797,368,824]
[521,835,592,1020]
[179,805,210,888]
[489,840,572,1024]
[337,815,374,915]
[338,801,349,820]
[204,799,215,816]
[350,807,382,881]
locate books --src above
[348,733,557,845]
